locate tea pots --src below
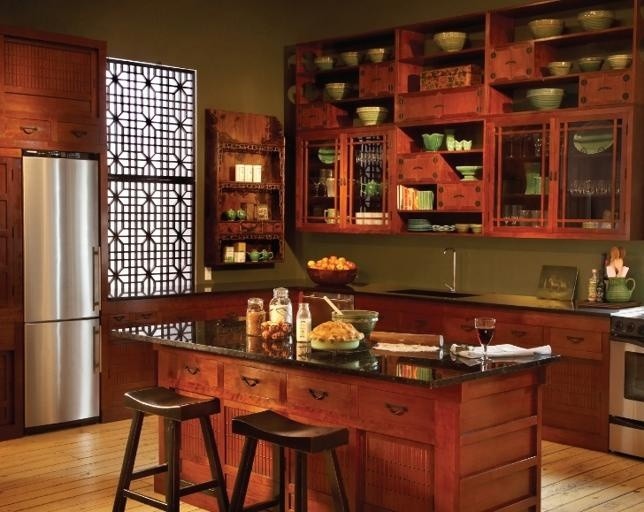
[246,249,274,263]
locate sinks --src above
[386,288,477,299]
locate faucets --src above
[442,246,459,295]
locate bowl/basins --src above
[340,51,363,65]
[332,310,379,344]
[455,223,470,232]
[547,61,572,75]
[578,9,614,30]
[314,56,335,70]
[307,267,358,287]
[366,47,389,63]
[324,81,352,100]
[528,18,562,38]
[469,224,482,232]
[578,56,604,71]
[526,88,565,109]
[607,54,630,68]
[356,106,389,126]
[329,348,380,372]
[434,31,466,51]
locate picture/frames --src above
[537,265,581,301]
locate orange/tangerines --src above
[308,255,354,271]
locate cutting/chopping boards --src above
[577,300,640,309]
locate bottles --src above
[295,342,312,362]
[587,269,599,302]
[245,287,312,342]
[245,336,263,355]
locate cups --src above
[324,209,339,225]
[519,210,547,227]
[569,179,620,195]
[474,317,496,363]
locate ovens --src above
[608,335,644,459]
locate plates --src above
[572,120,613,155]
[317,141,340,164]
[407,219,433,231]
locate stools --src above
[231,409,350,512]
[112,386,230,512]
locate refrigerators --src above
[23,149,102,433]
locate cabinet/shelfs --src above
[205,109,287,271]
[284,1,644,241]
[100,294,610,452]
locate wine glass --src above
[502,205,524,226]
[310,168,334,198]
